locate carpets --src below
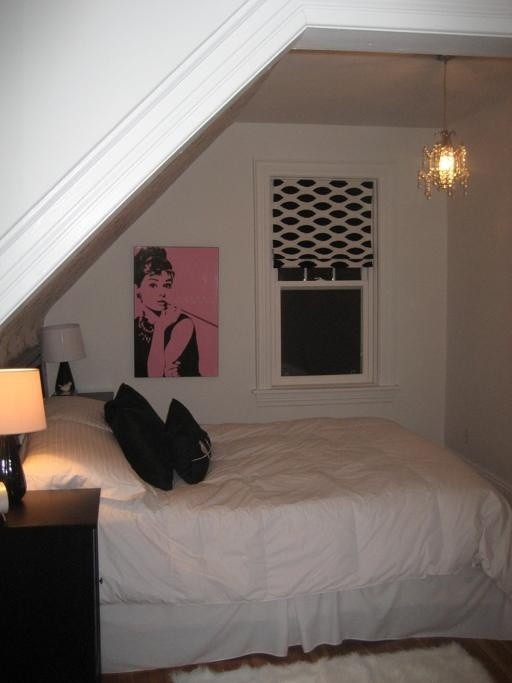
[168,634,493,682]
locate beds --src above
[4,344,510,677]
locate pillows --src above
[101,377,175,492]
[162,393,213,484]
[39,386,110,429]
[19,425,155,506]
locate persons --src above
[134,246,202,377]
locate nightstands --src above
[75,388,116,403]
[0,486,108,677]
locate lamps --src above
[416,54,471,199]
[39,319,88,387]
[0,359,51,507]
